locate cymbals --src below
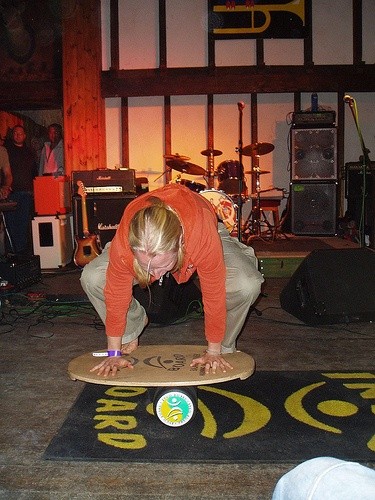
[245,170,272,175]
[240,142,276,156]
[200,149,223,157]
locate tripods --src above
[243,149,276,241]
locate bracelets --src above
[107,350,122,357]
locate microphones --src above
[237,101,245,109]
[343,95,354,103]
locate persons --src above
[0,135,13,264]
[3,125,38,253]
[30,124,64,176]
[80,183,265,377]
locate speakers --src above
[290,126,339,181]
[30,176,139,269]
[280,247,375,327]
[291,180,339,237]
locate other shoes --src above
[19,249,33,255]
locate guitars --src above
[74,179,102,268]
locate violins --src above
[162,153,207,175]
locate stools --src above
[252,200,282,234]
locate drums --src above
[197,189,237,235]
[177,178,206,194]
[217,159,249,182]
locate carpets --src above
[40,367,375,463]
